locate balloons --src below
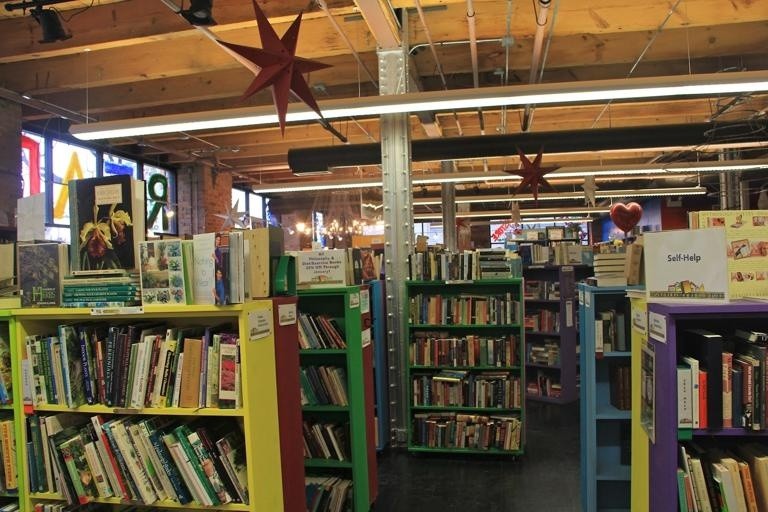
[610,202,642,232]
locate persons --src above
[213,267,225,304]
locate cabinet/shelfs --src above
[576,283,768,511]
[296,279,390,510]
[406,276,526,456]
[1,266,306,512]
[522,269,574,406]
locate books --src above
[676,325,767,511]
[283,246,383,288]
[1,173,284,308]
[408,234,522,451]
[2,319,242,409]
[292,314,355,511]
[1,412,249,511]
[594,307,632,465]
[590,210,768,303]
[507,225,595,399]
